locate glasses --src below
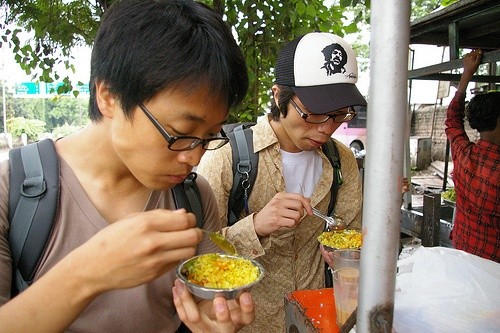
[288,97,356,125]
[137,102,230,150]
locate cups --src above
[329,249,359,329]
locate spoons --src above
[309,208,345,230]
[189,225,235,255]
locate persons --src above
[445,48,500,264]
[191,32,369,333]
[0,0,255,333]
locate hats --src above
[274,32,367,113]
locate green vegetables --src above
[441,188,456,202]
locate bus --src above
[331,104,368,170]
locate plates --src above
[316,230,365,254]
[175,253,267,300]
